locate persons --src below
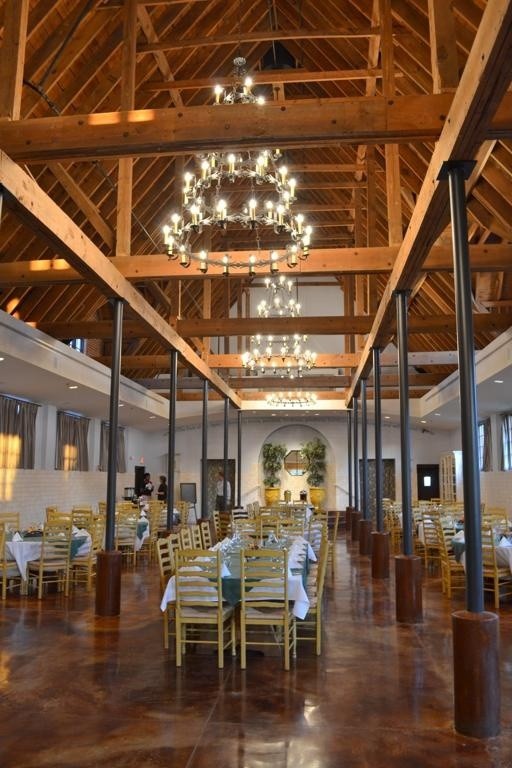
[215,472,231,513]
[155,475,167,504]
[140,473,154,496]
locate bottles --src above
[284,491,291,502]
[300,491,307,501]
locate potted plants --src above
[300,438,329,507]
[258,441,287,506]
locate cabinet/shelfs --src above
[438,450,464,499]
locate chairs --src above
[381,497,512,610]
[155,499,340,668]
[0,501,190,600]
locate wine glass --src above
[9,520,61,538]
[203,528,289,574]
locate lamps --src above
[236,270,320,378]
[267,390,318,407]
[161,56,314,279]
